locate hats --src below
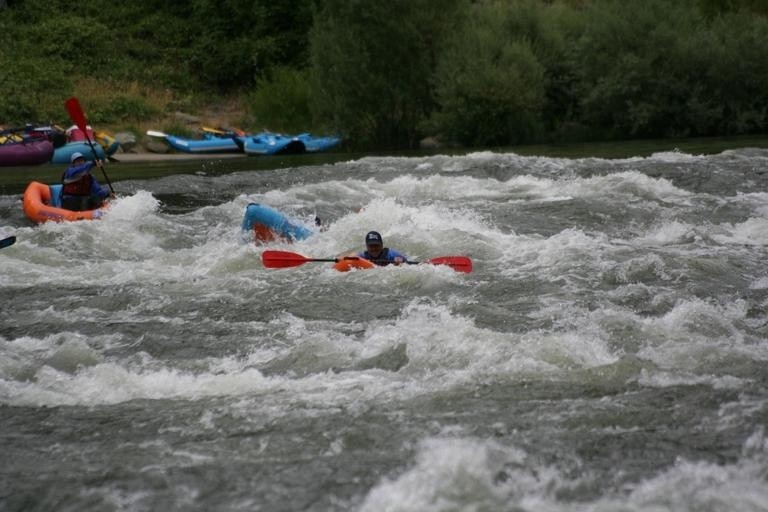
[71,152,85,162]
[366,231,382,245]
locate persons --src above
[59,152,116,211]
[337,230,407,265]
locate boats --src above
[164,131,342,156]
[0,129,106,165]
[24,181,109,225]
[334,255,375,273]
[243,203,312,246]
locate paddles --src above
[54,125,120,163]
[262,251,472,275]
[202,126,235,136]
[64,96,114,192]
[146,130,169,138]
